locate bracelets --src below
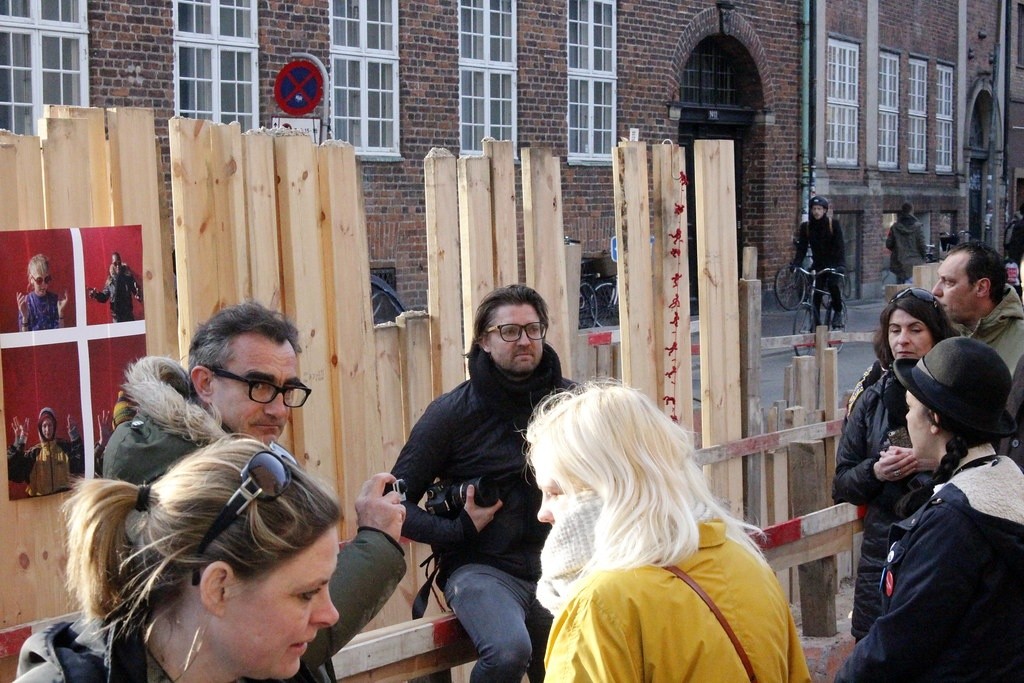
[59,317,64,319]
[22,323,28,327]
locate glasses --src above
[31,274,51,284]
[112,261,121,267]
[486,321,547,342]
[888,287,947,327]
[191,441,298,587]
[205,364,311,408]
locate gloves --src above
[837,265,845,274]
[790,264,800,274]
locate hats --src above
[112,392,136,430]
[894,337,1018,438]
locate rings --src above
[894,470,901,476]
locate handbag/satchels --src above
[886,223,896,249]
[1003,221,1018,250]
[1005,261,1020,286]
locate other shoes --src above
[831,311,842,325]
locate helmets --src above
[810,197,828,213]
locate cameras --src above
[382,478,407,502]
[425,475,501,521]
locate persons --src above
[885,201,930,285]
[515,378,814,683]
[16,254,68,332]
[790,195,845,333]
[94,390,137,478]
[9,434,344,683]
[7,407,84,497]
[104,303,406,683]
[86,252,143,323]
[383,284,590,683]
[832,203,1024,683]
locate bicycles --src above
[774,236,852,310]
[918,231,973,266]
[564,237,619,329]
[789,262,846,357]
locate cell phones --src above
[887,425,913,449]
